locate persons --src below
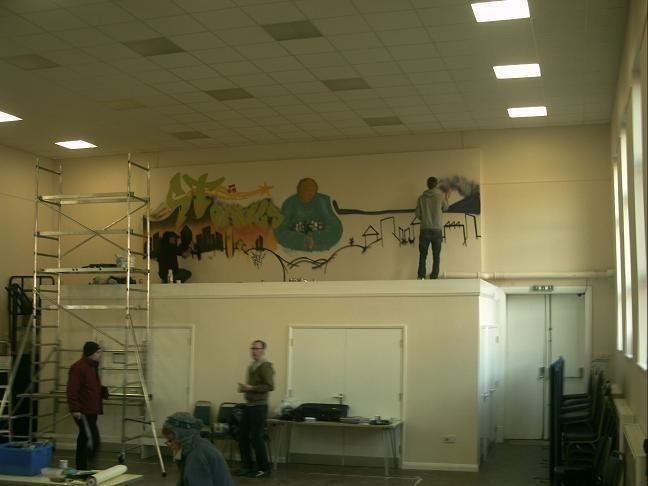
[152,232,191,284]
[414,177,449,280]
[67,340,105,470]
[163,410,231,486]
[235,340,276,478]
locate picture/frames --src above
[142,148,484,283]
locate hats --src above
[82,341,101,356]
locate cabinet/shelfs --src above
[264,416,403,476]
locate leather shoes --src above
[248,469,271,477]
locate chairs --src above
[211,403,239,468]
[194,400,211,443]
[263,423,273,467]
[549,357,616,486]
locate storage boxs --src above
[0,442,54,475]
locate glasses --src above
[250,347,264,350]
